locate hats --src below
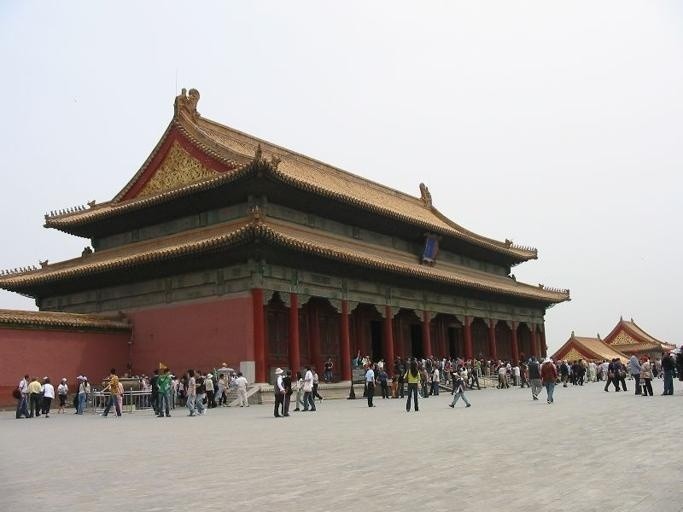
[275,367,283,374]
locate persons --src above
[603,352,674,395]
[511,356,557,403]
[466,355,510,390]
[450,372,471,408]
[556,358,608,388]
[366,363,377,407]
[15,368,250,419]
[357,353,398,399]
[396,354,468,399]
[323,357,334,383]
[274,366,324,417]
[403,361,422,411]
